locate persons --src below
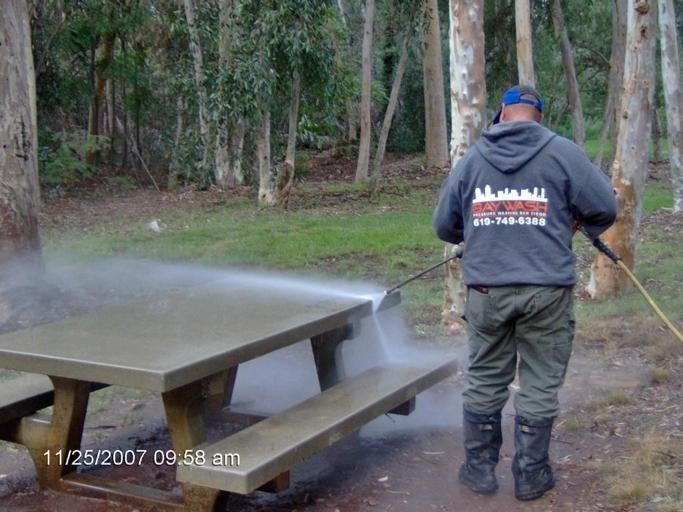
[432,84,615,499]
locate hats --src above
[493,84,543,124]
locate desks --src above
[0,280,459,512]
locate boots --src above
[459,410,502,494]
[510,415,555,501]
